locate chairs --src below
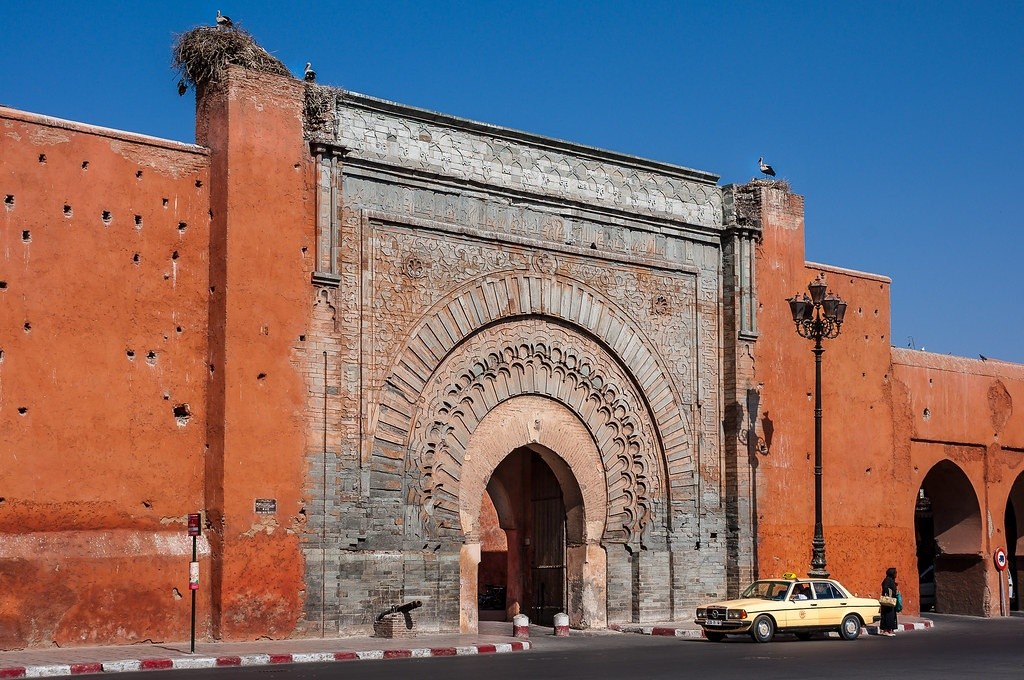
[823,587,838,598]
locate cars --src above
[694,571,882,643]
[918,562,1014,612]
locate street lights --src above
[784,273,849,577]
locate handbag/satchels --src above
[895,591,903,613]
[879,588,897,607]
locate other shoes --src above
[888,631,896,636]
[882,631,893,636]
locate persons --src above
[879,567,898,636]
[822,584,827,593]
[791,586,808,600]
